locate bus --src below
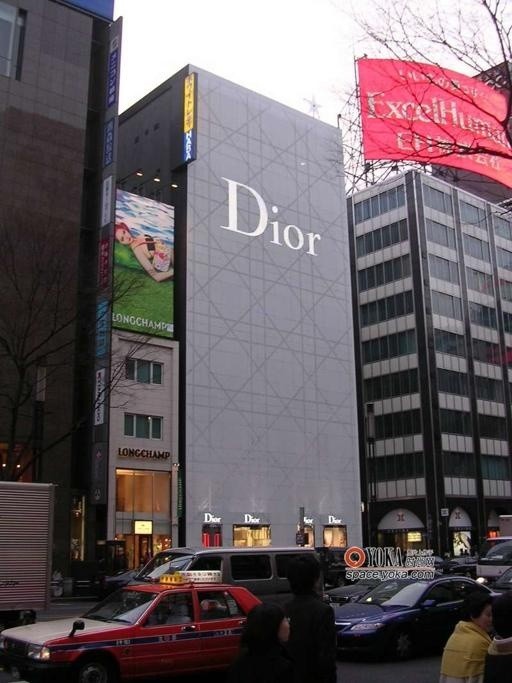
[477,537,512,578]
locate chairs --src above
[165,604,192,625]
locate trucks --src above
[0,481,55,625]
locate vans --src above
[128,545,325,610]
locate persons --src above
[484,590,512,683]
[201,601,227,620]
[285,552,338,683]
[115,222,174,283]
[144,548,151,566]
[233,603,294,682]
[51,567,65,597]
[112,547,128,576]
[439,590,493,682]
[96,547,114,602]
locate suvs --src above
[315,546,364,586]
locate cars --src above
[323,568,512,664]
[430,555,477,580]
[1,574,268,682]
